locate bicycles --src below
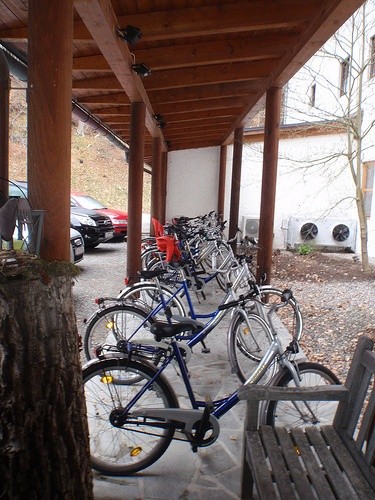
[82,287,344,476]
[82,209,304,386]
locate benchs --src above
[237,335,375,500]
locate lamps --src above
[132,63,152,77]
[154,113,167,128]
[116,24,143,47]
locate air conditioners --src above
[241,214,259,240]
[281,216,357,252]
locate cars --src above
[0,178,115,265]
[71,192,127,242]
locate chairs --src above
[152,218,181,261]
